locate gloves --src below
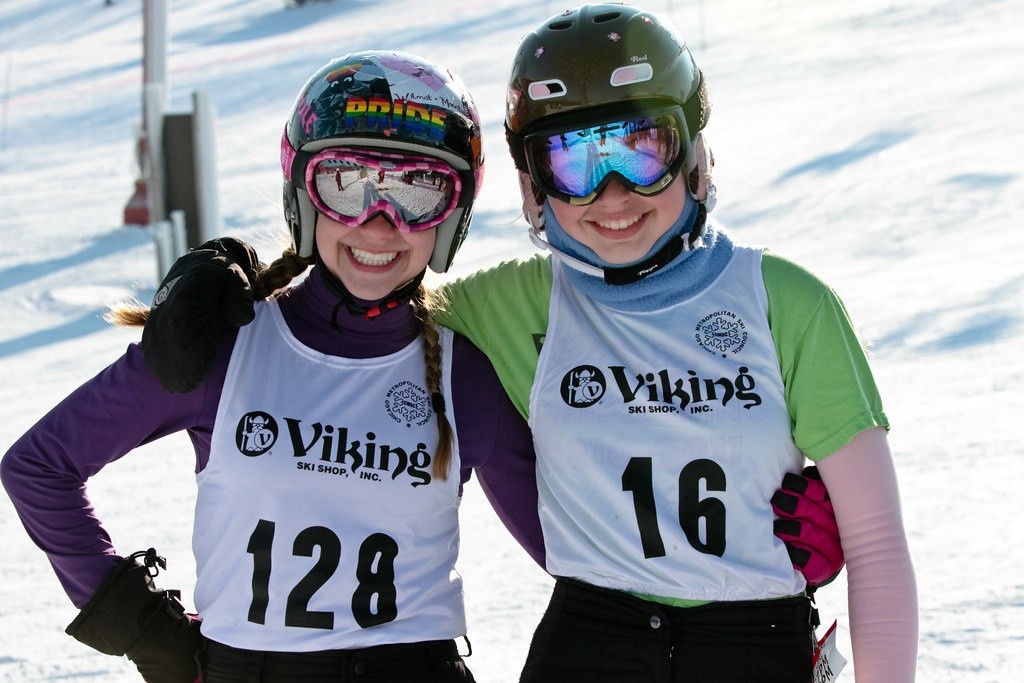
[770,468,846,604]
[66,548,204,683]
[141,235,259,393]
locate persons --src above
[0,49,846,683]
[141,3,920,683]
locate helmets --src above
[504,3,710,172]
[279,49,487,274]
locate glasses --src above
[523,104,691,205]
[304,146,461,234]
